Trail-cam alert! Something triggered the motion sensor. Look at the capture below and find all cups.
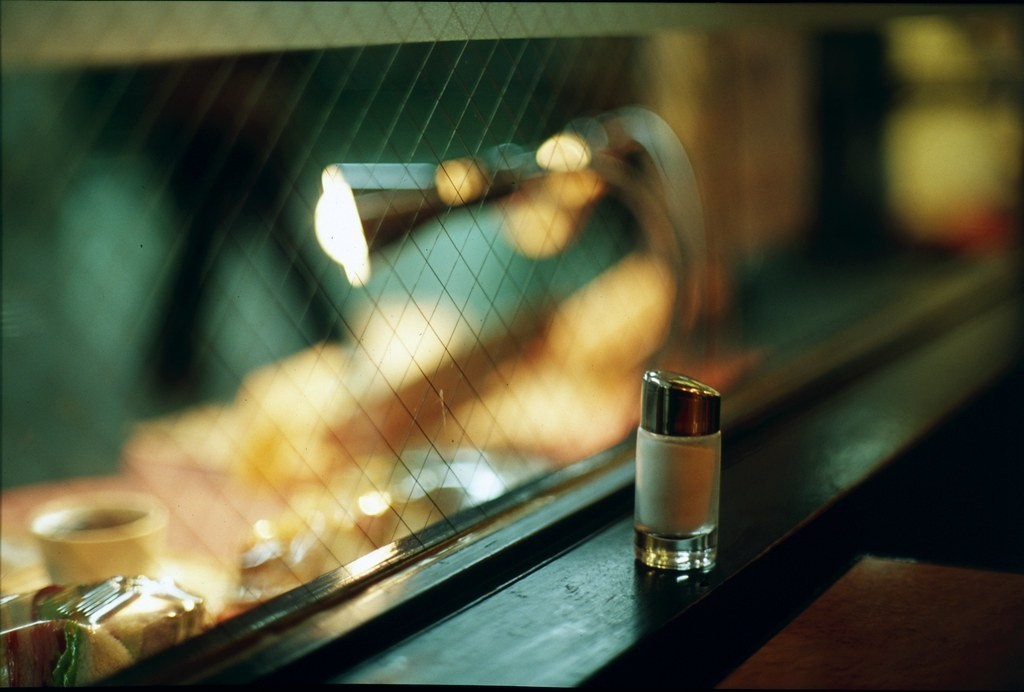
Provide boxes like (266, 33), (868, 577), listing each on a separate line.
(31, 506), (162, 586)
